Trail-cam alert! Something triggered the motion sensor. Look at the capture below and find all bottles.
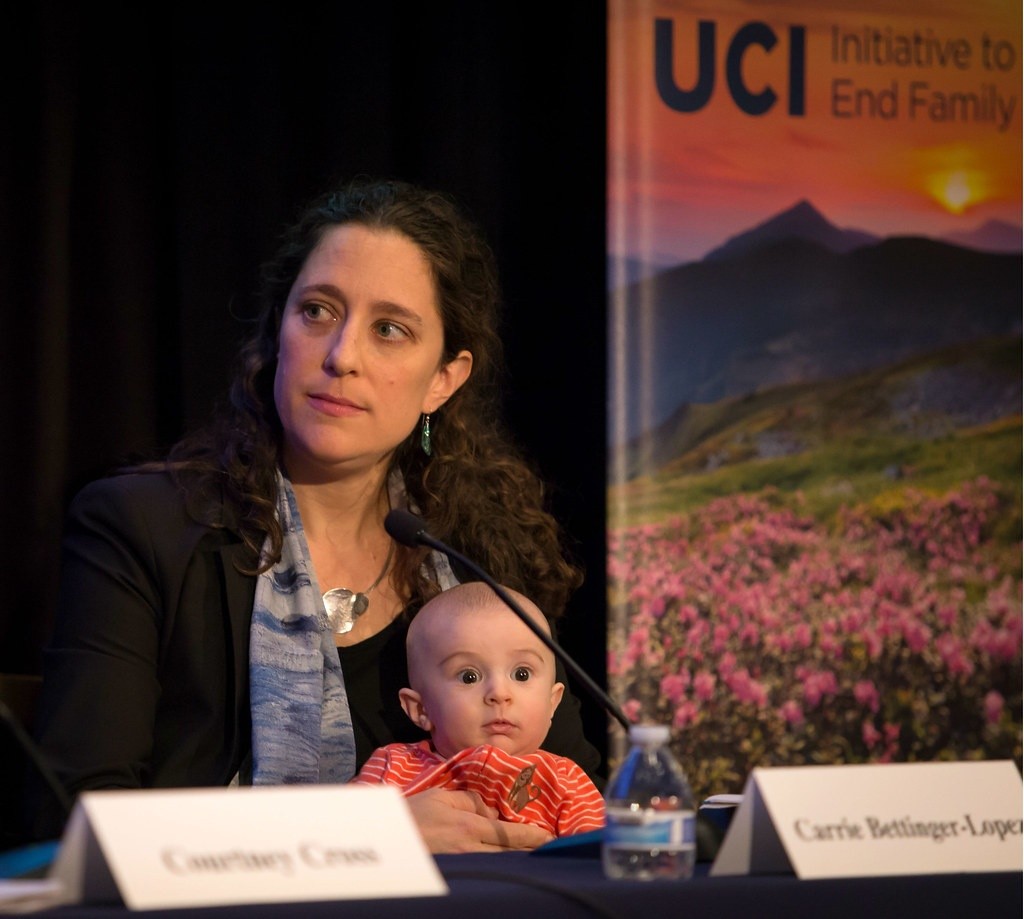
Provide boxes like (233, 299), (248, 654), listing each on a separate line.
(604, 724), (696, 883)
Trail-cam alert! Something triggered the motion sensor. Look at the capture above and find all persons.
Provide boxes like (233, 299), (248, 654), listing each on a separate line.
(345, 582), (607, 852)
(47, 180), (601, 806)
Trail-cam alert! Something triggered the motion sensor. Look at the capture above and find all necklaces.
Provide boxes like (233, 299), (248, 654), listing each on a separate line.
(322, 535), (407, 636)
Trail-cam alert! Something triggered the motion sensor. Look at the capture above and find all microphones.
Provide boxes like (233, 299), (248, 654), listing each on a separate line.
(382, 508), (719, 865)
